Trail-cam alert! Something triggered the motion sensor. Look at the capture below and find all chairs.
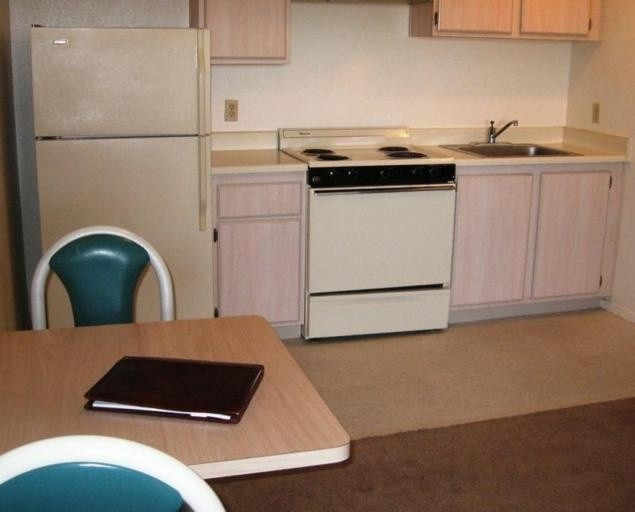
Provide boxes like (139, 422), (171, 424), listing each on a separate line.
(30, 225), (174, 331)
(0, 435), (232, 512)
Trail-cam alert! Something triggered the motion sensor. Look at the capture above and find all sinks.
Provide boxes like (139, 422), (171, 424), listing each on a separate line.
(438, 143), (584, 159)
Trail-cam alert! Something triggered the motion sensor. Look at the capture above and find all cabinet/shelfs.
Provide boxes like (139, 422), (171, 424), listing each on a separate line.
(408, 0), (603, 43)
(451, 160), (624, 310)
(211, 170), (305, 338)
(190, 0), (291, 65)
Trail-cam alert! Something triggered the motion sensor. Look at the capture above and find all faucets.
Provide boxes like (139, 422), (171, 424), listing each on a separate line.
(488, 119), (518, 144)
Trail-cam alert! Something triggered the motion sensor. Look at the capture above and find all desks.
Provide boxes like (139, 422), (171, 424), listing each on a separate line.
(0, 315), (351, 479)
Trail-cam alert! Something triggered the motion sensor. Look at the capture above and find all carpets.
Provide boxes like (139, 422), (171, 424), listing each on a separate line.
(181, 399), (635, 512)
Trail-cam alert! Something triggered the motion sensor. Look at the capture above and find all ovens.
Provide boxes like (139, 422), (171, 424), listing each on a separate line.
(304, 185), (456, 342)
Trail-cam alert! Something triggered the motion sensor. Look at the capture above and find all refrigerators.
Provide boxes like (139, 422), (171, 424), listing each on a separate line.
(29, 26), (215, 332)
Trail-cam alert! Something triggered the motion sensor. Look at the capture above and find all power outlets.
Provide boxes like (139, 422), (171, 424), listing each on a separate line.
(225, 99), (238, 122)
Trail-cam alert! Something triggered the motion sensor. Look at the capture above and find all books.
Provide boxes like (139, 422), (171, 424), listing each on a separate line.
(83, 355), (264, 424)
